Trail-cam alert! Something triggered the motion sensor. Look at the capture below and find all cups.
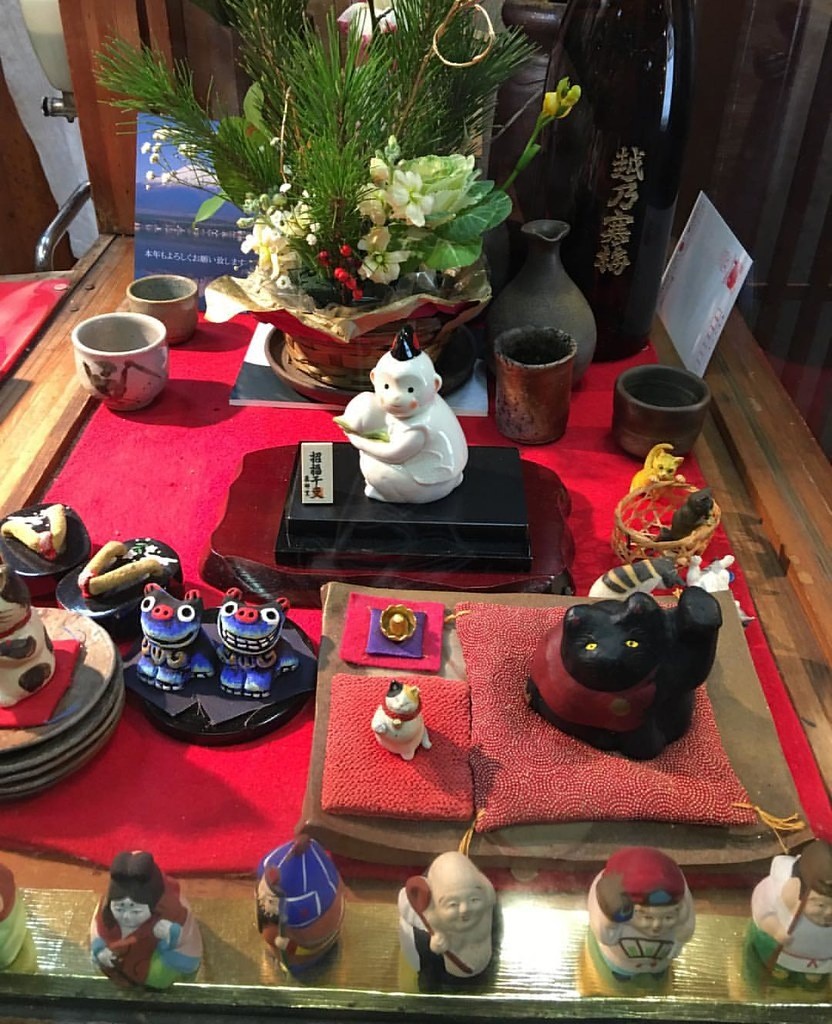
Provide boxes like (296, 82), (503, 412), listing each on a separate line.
(72, 313), (170, 412)
(613, 365), (709, 461)
(494, 329), (577, 445)
(126, 274), (199, 346)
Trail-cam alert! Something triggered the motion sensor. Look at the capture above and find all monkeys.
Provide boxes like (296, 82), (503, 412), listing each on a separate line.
(331, 324), (469, 505)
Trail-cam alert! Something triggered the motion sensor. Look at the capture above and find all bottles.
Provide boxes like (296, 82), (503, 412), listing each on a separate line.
(485, 220), (597, 385)
(539, 0), (692, 361)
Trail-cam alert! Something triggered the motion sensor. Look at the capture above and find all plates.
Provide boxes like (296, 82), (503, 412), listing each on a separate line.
(0, 609), (126, 802)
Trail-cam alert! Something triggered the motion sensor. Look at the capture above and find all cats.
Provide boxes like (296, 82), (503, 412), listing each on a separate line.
(588, 442), (756, 635)
(524, 585), (722, 761)
(0, 563), (57, 710)
(370, 678), (433, 761)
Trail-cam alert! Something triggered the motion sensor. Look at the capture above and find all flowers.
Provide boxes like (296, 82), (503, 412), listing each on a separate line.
(84, 0), (581, 306)
